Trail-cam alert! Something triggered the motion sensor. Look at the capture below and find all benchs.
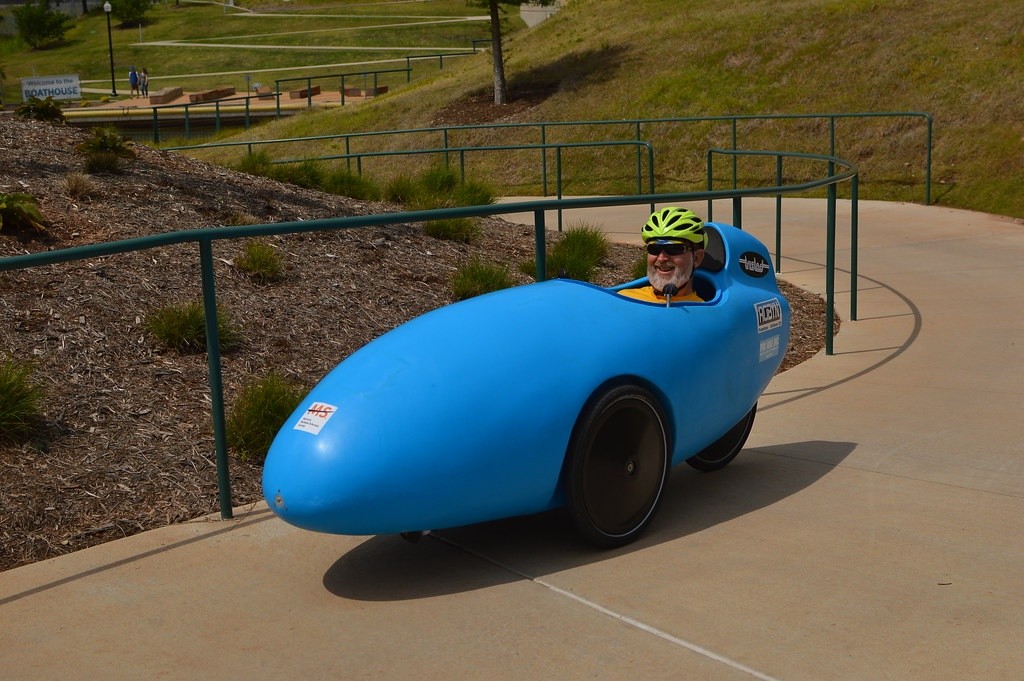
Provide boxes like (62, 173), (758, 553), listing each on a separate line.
(148, 86), (387, 106)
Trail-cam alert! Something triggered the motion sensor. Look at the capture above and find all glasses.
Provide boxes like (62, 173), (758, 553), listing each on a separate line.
(644, 243), (696, 256)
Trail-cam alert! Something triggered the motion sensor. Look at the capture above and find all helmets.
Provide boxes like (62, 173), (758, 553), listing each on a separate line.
(640, 206), (709, 251)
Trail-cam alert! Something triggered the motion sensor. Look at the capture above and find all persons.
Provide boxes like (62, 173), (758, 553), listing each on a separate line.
(128, 65), (148, 99)
(615, 206), (708, 304)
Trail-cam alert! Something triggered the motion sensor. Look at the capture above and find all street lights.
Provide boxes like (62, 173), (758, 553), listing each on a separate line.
(102, 1), (118, 96)
